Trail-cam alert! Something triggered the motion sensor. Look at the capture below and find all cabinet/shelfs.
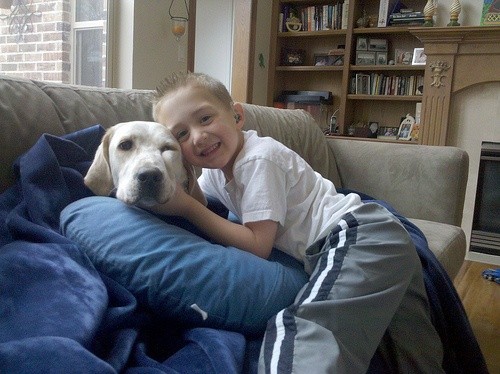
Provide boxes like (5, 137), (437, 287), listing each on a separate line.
(267, 0), (427, 144)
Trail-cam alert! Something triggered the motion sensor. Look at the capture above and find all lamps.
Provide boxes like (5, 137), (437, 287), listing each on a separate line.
(168, 0), (190, 42)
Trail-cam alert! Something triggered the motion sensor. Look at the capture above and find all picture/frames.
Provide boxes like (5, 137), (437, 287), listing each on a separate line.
(396, 113), (415, 141)
(412, 48), (427, 66)
(356, 74), (370, 95)
(313, 54), (330, 65)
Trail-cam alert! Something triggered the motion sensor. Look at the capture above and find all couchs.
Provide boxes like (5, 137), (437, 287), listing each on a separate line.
(0, 78), (466, 284)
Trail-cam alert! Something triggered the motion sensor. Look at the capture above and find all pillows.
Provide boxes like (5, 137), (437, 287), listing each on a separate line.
(59, 194), (308, 333)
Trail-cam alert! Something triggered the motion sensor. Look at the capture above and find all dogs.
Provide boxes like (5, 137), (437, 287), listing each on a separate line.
(83, 120), (209, 210)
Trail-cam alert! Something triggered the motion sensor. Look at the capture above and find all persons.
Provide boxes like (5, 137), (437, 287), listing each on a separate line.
(149, 69), (445, 374)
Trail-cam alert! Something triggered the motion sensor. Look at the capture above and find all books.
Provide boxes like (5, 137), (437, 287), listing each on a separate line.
(276, 2), (427, 130)
